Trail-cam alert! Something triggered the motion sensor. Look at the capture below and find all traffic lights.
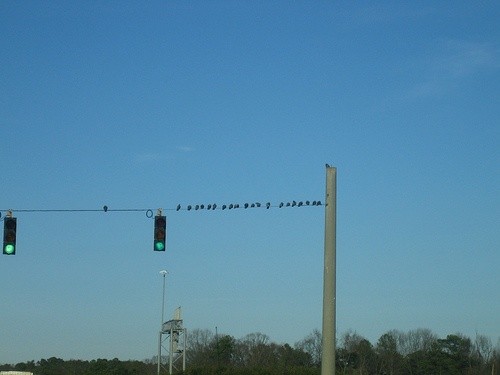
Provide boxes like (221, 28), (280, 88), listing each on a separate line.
(154, 216), (166, 250)
(3, 217), (16, 254)
